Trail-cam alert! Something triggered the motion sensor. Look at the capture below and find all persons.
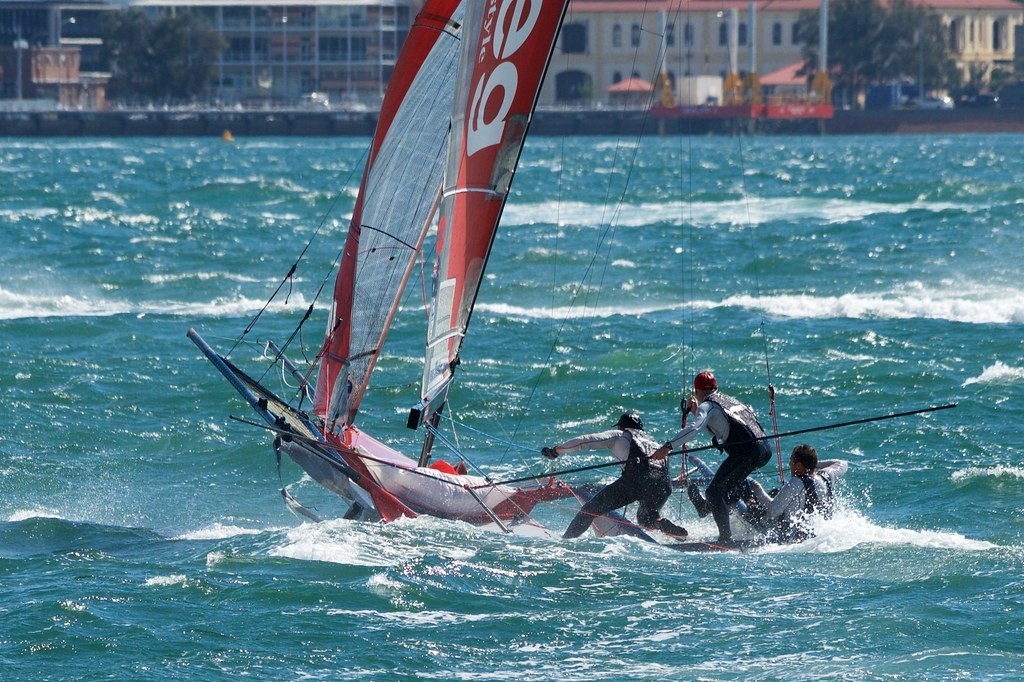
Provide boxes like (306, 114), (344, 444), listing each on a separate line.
(651, 372), (772, 548)
(541, 413), (688, 542)
(688, 444), (848, 545)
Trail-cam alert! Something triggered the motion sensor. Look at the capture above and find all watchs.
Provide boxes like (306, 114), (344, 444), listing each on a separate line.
(664, 442), (674, 450)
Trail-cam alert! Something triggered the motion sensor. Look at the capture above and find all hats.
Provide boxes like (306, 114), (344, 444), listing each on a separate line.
(611, 413), (645, 431)
(694, 371), (718, 390)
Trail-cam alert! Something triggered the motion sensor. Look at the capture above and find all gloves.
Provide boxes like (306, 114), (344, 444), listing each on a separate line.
(541, 446), (559, 460)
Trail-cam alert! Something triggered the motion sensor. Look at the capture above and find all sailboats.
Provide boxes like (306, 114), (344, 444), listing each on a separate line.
(185, 0), (847, 553)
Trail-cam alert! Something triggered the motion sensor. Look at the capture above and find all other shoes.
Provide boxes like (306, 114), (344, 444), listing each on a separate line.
(688, 484), (711, 518)
(659, 519), (688, 543)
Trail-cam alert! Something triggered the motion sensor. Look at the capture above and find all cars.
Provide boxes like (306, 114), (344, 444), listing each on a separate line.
(302, 94), (330, 109)
(906, 96), (958, 112)
